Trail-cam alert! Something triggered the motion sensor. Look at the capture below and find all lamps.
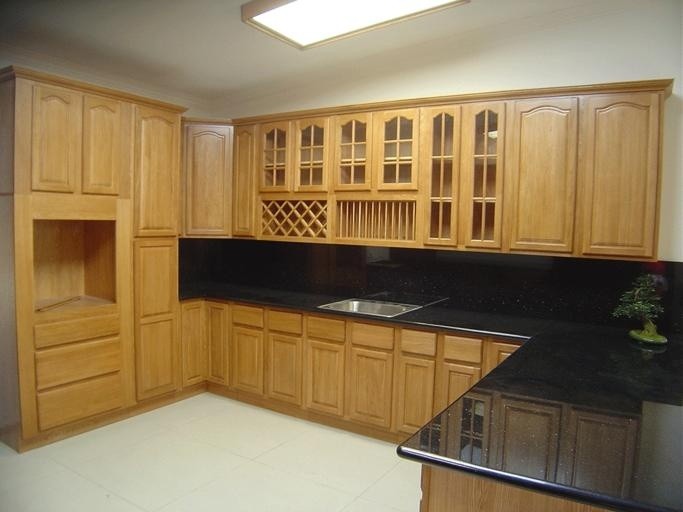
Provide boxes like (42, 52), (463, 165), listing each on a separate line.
(240, 1), (472, 52)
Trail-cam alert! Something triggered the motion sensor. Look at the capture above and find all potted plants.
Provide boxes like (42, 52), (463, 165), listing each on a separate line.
(613, 272), (670, 345)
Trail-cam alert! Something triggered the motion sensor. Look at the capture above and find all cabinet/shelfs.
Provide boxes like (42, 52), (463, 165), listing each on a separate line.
(395, 328), (441, 434)
(490, 340), (518, 372)
(262, 94), (500, 249)
(31, 84), (78, 192)
(177, 302), (204, 391)
(130, 103), (178, 238)
(417, 389), (492, 468)
(134, 238), (184, 401)
(504, 91), (661, 257)
(181, 118), (233, 242)
(438, 337), (485, 414)
(347, 325), (396, 432)
(211, 299), (229, 390)
(305, 317), (347, 422)
(229, 304), (266, 394)
(82, 90), (117, 193)
(229, 118), (257, 236)
(265, 307), (304, 412)
(495, 397), (638, 494)
(37, 219), (119, 440)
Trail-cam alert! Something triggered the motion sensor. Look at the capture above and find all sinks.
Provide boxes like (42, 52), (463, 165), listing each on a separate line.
(365, 291), (449, 307)
(318, 297), (424, 319)
